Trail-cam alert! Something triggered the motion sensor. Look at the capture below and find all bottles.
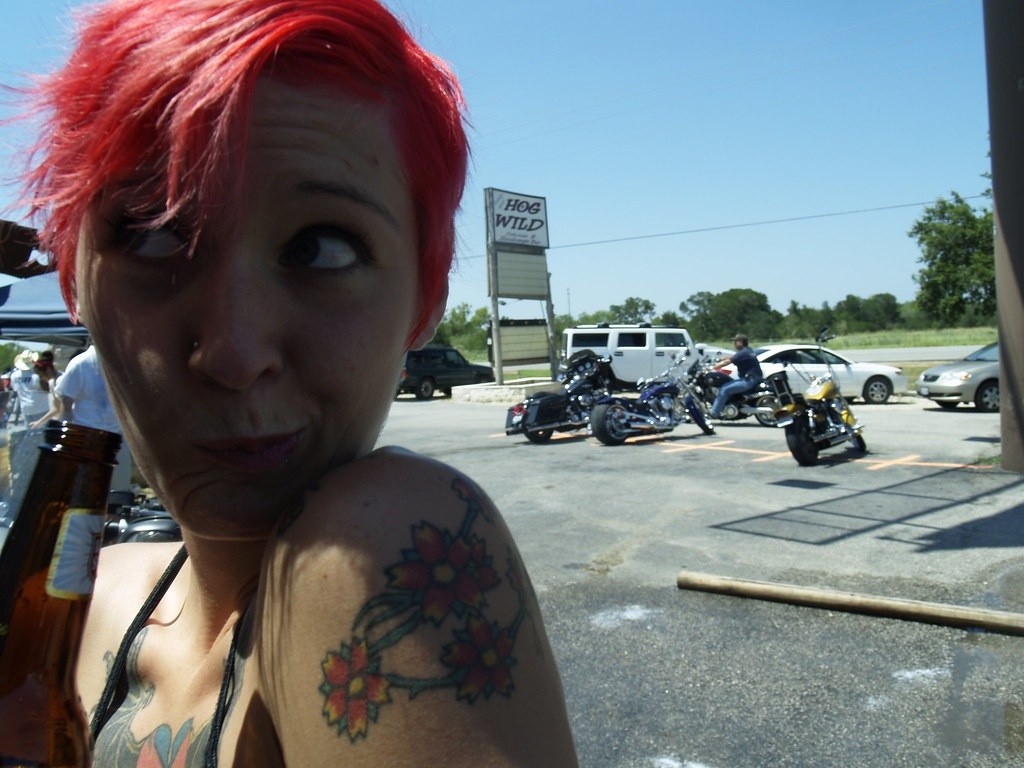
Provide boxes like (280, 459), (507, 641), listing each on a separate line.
(0, 420), (124, 768)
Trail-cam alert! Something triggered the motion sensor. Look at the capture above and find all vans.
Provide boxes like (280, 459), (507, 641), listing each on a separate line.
(556, 321), (704, 393)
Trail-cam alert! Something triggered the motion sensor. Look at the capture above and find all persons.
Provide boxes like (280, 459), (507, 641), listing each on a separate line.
(0, 330), (763, 492)
(0, 0), (576, 768)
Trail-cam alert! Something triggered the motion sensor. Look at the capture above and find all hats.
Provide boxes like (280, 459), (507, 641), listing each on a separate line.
(13, 350), (39, 371)
(731, 335), (747, 342)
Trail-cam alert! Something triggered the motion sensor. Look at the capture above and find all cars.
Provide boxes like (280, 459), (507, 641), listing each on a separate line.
(914, 340), (1002, 413)
(714, 342), (909, 406)
(696, 342), (738, 368)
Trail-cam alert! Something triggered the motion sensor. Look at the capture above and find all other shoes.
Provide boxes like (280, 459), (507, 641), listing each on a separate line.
(703, 408), (714, 419)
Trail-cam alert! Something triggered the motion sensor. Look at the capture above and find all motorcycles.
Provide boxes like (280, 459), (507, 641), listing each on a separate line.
(591, 348), (716, 444)
(776, 328), (868, 466)
(681, 349), (781, 428)
(504, 347), (615, 445)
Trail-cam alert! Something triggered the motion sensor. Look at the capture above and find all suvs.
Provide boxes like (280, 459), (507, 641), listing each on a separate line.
(394, 345), (495, 400)
